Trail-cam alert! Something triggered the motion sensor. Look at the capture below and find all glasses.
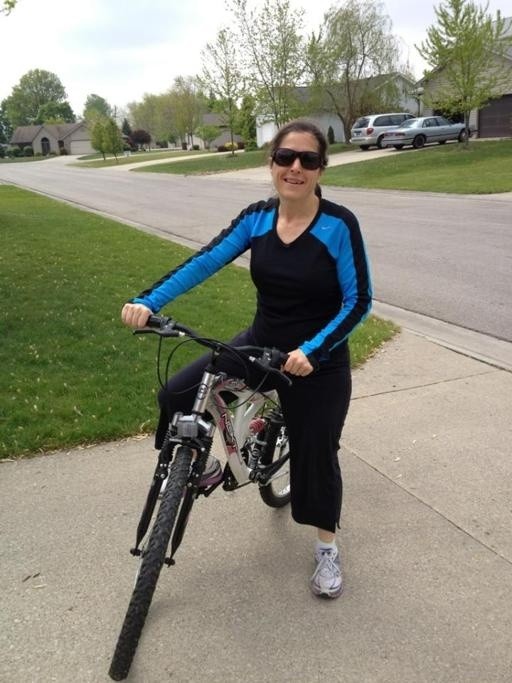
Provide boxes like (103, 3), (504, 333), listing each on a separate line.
(272, 148), (322, 170)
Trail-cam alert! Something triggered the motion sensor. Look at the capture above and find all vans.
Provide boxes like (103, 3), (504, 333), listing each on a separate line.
(348, 112), (416, 150)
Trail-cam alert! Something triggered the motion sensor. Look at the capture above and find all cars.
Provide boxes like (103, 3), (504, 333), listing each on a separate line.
(381, 115), (472, 149)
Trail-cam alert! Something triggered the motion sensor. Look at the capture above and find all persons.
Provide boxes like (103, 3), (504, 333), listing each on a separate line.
(118, 114), (375, 600)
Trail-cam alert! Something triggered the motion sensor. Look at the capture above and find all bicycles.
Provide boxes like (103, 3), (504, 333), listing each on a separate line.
(108, 313), (292, 682)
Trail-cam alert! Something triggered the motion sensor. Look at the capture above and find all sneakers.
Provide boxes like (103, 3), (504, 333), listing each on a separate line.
(310, 546), (343, 600)
(158, 456), (222, 500)
